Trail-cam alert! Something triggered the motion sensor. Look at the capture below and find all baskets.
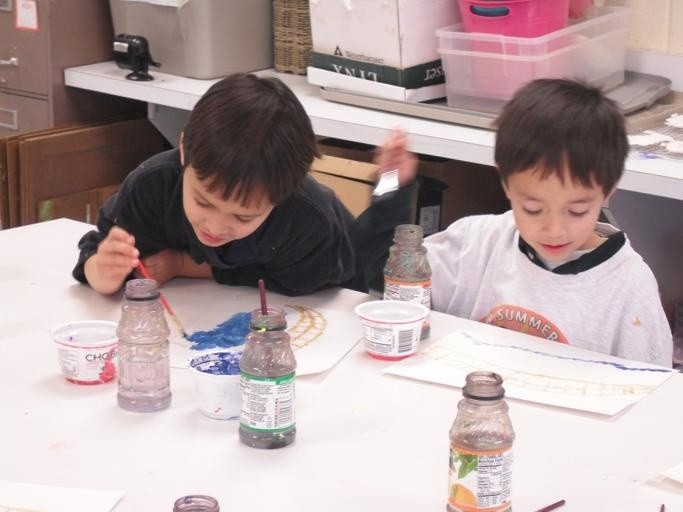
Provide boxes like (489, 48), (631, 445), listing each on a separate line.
(273, 1), (313, 74)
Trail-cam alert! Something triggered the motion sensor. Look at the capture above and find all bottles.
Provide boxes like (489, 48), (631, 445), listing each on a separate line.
(383, 222), (434, 342)
(446, 369), (518, 512)
(117, 277), (173, 413)
(237, 306), (299, 450)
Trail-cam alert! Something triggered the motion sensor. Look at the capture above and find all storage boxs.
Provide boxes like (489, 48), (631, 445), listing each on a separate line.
(109, 0), (274, 80)
(307, 137), (450, 235)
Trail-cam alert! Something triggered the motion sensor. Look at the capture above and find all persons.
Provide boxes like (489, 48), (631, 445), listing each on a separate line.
(351, 79), (672, 367)
(73, 72), (368, 296)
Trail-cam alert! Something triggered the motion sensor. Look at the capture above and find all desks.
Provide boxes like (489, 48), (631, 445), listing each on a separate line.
(0, 216), (683, 511)
(62, 59), (683, 201)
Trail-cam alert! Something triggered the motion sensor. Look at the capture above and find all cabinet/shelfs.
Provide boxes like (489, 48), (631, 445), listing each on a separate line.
(0, 0), (130, 135)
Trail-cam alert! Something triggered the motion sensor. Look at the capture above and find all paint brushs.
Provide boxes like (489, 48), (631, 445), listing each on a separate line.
(137, 259), (190, 343)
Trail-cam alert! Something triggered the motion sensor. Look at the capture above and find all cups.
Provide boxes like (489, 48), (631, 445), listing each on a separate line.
(190, 350), (250, 420)
(52, 318), (120, 385)
(354, 297), (429, 359)
